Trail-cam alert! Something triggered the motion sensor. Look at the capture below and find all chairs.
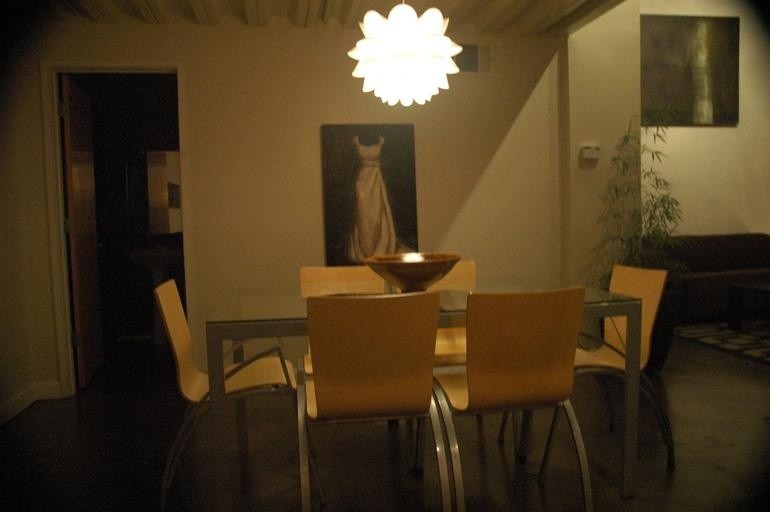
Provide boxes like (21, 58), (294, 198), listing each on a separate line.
(299, 261), (477, 316)
(498, 263), (678, 488)
(295, 289), (452, 510)
(432, 286), (596, 509)
(154, 277), (328, 510)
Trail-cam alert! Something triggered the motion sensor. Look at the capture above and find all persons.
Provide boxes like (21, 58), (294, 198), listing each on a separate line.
(335, 127), (416, 264)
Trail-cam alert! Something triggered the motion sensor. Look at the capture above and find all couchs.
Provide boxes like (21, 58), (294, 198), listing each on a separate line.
(641, 233), (770, 321)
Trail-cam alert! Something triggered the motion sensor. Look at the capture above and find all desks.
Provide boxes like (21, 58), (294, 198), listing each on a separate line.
(206, 285), (641, 508)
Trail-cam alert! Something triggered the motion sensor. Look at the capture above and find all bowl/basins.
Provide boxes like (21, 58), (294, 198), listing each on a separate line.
(367, 252), (462, 295)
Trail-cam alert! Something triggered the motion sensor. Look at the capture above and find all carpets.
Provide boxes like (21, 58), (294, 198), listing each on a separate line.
(674, 320), (770, 366)
